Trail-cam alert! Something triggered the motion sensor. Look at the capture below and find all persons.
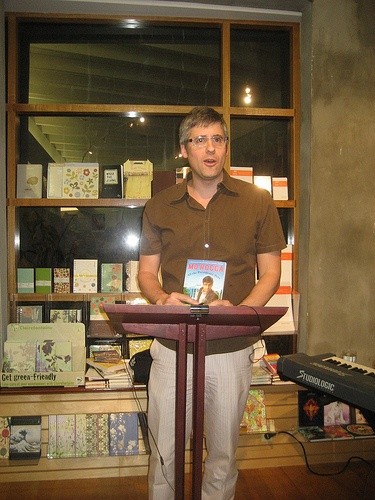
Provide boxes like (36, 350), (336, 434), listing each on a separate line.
(136, 105), (283, 500)
(193, 275), (219, 305)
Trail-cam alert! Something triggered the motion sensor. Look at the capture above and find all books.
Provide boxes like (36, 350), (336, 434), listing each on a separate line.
(0, 243), (294, 461)
(16, 159), (289, 201)
(182, 258), (229, 306)
(296, 389), (375, 442)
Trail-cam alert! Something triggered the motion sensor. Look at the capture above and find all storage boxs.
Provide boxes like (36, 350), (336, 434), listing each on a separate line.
(17, 161), (293, 294)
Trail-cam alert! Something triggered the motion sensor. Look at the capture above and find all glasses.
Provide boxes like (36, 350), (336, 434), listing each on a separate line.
(184, 135), (228, 148)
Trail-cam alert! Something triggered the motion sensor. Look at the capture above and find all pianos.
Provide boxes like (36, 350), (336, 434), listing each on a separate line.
(277, 353), (375, 433)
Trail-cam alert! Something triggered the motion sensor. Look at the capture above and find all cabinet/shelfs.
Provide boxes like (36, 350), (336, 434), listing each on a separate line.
(4, 9), (304, 393)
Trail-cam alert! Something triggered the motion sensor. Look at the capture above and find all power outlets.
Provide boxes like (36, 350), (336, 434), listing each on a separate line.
(263, 432), (274, 443)
